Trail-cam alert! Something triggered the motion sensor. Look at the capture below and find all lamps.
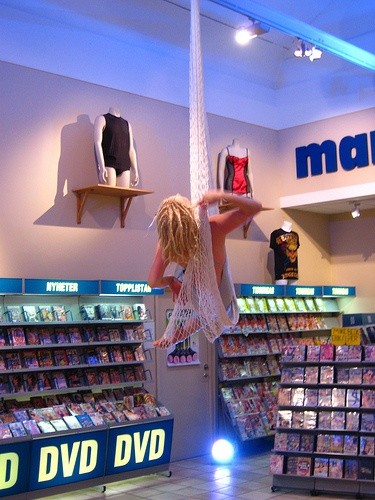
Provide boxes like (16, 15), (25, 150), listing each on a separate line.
(294, 47), (323, 62)
(235, 22), (270, 45)
(351, 203), (361, 219)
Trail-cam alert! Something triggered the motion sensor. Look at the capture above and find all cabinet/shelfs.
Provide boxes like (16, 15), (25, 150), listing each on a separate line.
(0, 296), (375, 500)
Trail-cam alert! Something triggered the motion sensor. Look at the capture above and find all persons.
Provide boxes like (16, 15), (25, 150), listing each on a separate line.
(148, 191), (263, 348)
(219, 140), (254, 205)
(94, 106), (139, 188)
(270, 221), (301, 285)
